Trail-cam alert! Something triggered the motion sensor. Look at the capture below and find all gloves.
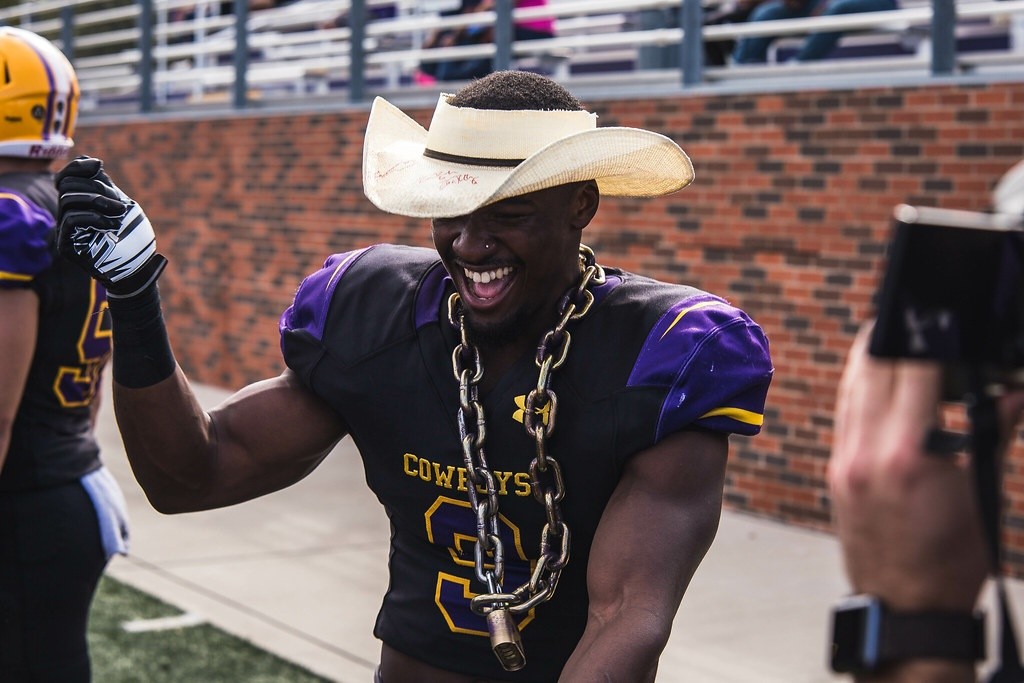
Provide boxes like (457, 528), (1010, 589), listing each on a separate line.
(54, 154), (166, 298)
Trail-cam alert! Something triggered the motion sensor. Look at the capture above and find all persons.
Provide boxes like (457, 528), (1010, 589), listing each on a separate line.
(830, 158), (1024, 683)
(53, 70), (771, 683)
(169, 0), (900, 84)
(0, 25), (131, 683)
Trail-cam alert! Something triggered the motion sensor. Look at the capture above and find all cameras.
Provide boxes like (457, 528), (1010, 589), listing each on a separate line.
(867, 204), (1024, 405)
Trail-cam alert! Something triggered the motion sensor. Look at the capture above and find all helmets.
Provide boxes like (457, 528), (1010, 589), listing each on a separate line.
(0, 22), (80, 160)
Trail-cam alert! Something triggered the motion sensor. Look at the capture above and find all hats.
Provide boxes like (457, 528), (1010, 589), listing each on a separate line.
(363, 92), (694, 218)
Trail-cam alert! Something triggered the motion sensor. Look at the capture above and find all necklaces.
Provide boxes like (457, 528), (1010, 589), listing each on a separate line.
(440, 243), (606, 671)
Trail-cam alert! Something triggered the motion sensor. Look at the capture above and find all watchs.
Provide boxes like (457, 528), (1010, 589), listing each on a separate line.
(831, 596), (986, 675)
